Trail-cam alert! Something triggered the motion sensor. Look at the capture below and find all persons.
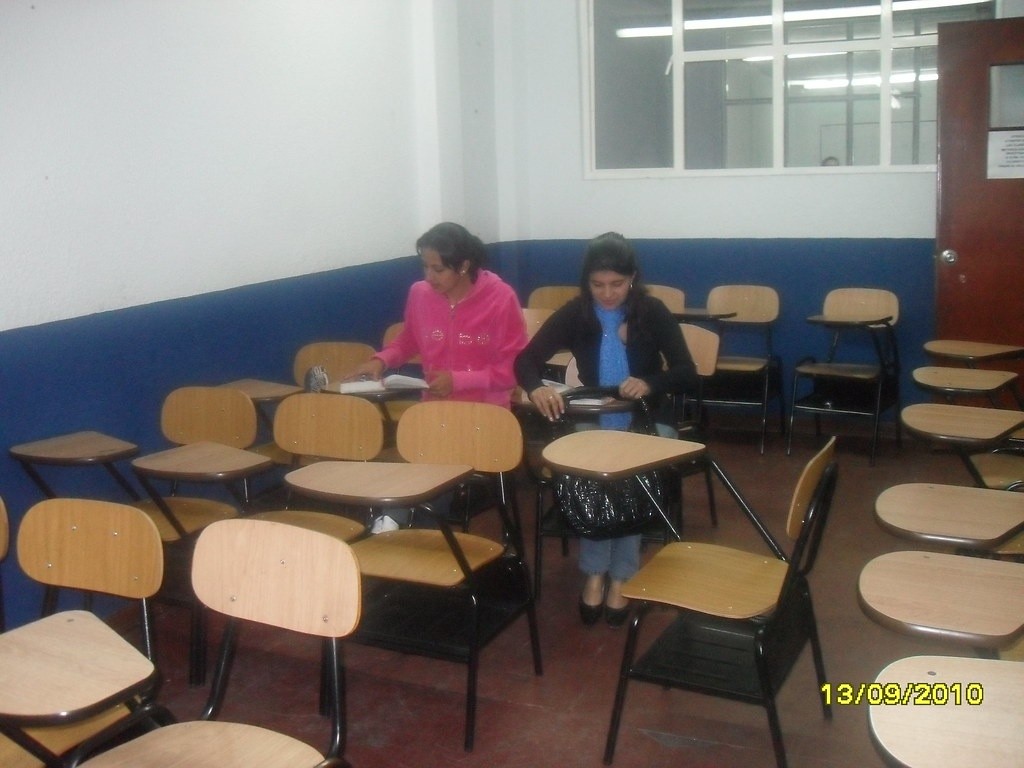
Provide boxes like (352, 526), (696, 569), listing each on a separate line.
(344, 223), (529, 535)
(514, 231), (697, 629)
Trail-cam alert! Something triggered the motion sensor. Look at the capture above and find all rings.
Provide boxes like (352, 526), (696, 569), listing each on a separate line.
(549, 396), (553, 399)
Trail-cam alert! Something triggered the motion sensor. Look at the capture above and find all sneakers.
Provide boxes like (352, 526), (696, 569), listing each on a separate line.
(371, 515), (400, 536)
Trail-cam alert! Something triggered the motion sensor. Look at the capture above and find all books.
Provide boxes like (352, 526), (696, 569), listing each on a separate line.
(341, 375), (431, 394)
(540, 378), (615, 406)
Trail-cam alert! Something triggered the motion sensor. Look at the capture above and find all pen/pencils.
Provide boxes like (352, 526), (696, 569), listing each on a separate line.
(360, 373), (366, 382)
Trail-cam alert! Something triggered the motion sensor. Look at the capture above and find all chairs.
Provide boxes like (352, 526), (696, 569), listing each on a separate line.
(0, 277), (1023, 768)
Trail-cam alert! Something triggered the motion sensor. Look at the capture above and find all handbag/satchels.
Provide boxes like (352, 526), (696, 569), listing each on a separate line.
(551, 385), (681, 541)
(669, 419), (716, 469)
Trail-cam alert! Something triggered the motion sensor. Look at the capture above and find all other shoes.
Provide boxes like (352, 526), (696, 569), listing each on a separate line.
(578, 584), (606, 626)
(606, 586), (632, 629)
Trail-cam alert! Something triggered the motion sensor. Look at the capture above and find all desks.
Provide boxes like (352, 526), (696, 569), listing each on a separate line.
(541, 428), (707, 479)
(664, 304), (739, 322)
(129, 439), (277, 483)
(283, 459), (475, 508)
(512, 392), (635, 414)
(901, 403), (1023, 450)
(213, 375), (304, 403)
(71, 719), (351, 768)
(921, 339), (1024, 363)
(855, 548), (1024, 649)
(0, 606), (164, 728)
(864, 654), (1024, 767)
(806, 316), (892, 325)
(869, 482), (1024, 549)
(318, 375), (422, 401)
(10, 428), (138, 464)
(910, 365), (1020, 396)
